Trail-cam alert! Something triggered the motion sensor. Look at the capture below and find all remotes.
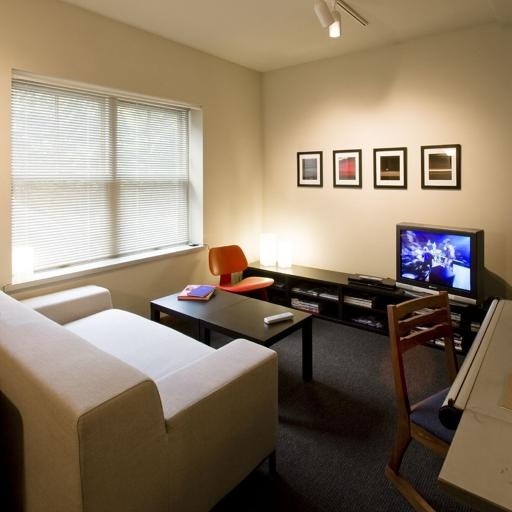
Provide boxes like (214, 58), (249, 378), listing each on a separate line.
(264, 312), (294, 325)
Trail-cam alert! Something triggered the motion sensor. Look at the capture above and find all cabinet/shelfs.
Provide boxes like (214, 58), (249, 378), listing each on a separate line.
(241, 257), (490, 356)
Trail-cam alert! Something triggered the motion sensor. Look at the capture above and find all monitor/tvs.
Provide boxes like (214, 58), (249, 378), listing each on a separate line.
(396, 222), (484, 305)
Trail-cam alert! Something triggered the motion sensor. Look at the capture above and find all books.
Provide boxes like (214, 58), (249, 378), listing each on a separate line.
(177, 284), (216, 301)
(187, 285), (216, 298)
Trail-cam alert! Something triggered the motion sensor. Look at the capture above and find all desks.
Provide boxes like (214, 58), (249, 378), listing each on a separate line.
(437, 298), (512, 511)
(149, 287), (316, 383)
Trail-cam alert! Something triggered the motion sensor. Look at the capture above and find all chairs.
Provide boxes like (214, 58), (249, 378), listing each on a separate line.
(383, 288), (461, 512)
(2, 280), (285, 512)
(207, 243), (276, 303)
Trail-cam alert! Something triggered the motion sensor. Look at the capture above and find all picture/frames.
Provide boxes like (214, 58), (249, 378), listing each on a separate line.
(372, 147), (408, 191)
(296, 150), (324, 188)
(419, 144), (462, 192)
(332, 149), (363, 189)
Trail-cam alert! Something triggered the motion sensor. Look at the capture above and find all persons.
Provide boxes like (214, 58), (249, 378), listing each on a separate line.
(420, 246), (434, 270)
(442, 240), (455, 266)
